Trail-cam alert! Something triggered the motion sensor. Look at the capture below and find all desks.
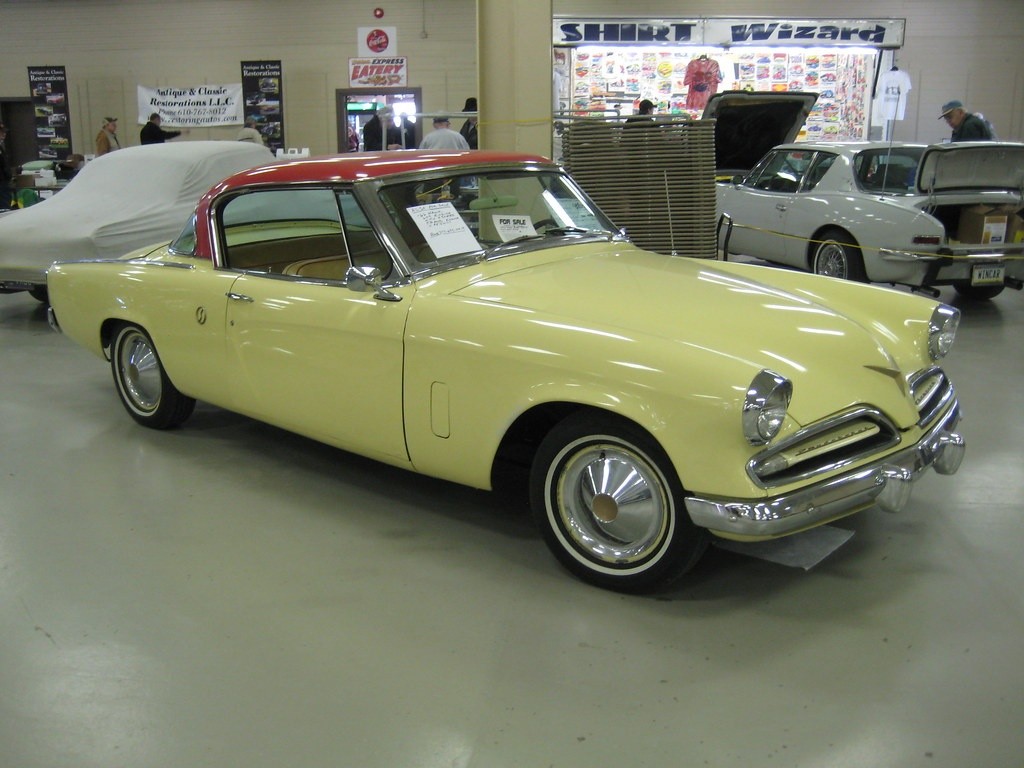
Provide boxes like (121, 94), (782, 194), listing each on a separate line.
(6, 175), (73, 203)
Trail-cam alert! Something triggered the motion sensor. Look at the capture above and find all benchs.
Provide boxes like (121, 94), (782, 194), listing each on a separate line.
(228, 233), (488, 281)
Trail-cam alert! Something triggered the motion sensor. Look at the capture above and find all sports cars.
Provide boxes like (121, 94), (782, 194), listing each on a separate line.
(45, 147), (966, 599)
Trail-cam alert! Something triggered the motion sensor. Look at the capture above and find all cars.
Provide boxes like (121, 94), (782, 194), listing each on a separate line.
(247, 77), (282, 151)
(34, 83), (69, 158)
(700, 88), (1024, 301)
(0, 138), (333, 306)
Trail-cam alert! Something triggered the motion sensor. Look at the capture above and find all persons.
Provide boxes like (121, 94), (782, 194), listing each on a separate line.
(238, 116), (265, 145)
(140, 113), (181, 145)
(96, 117), (121, 157)
(620, 100), (664, 143)
(0, 123), (11, 182)
(938, 101), (999, 144)
(364, 98), (479, 194)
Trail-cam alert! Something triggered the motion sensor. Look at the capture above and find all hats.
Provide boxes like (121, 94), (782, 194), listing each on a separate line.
(462, 97), (477, 112)
(245, 117), (255, 124)
(433, 111), (449, 121)
(639, 99), (657, 108)
(938, 100), (963, 119)
(102, 117), (118, 128)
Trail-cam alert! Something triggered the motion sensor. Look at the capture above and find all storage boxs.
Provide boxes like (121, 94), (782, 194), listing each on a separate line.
(956, 205), (1008, 244)
(986, 204), (1024, 244)
